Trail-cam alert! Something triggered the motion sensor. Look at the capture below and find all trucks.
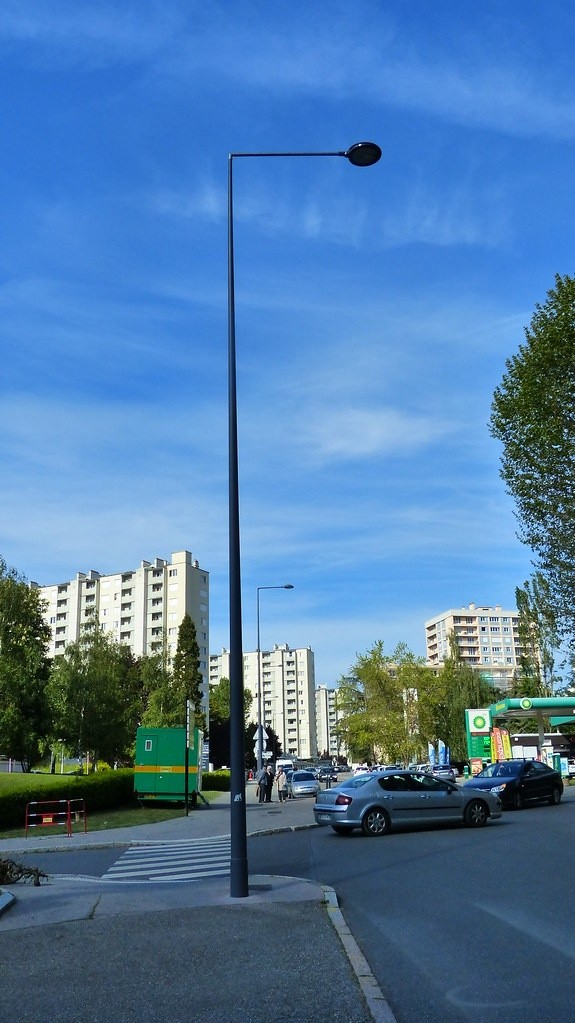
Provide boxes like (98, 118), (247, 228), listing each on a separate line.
(275, 759), (296, 775)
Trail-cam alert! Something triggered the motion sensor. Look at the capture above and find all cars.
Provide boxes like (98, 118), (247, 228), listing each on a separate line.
(287, 767), (318, 780)
(463, 757), (563, 810)
(319, 767), (337, 782)
(290, 772), (321, 798)
(334, 766), (350, 772)
(313, 770), (502, 836)
(351, 767), (370, 776)
(426, 764), (455, 782)
(371, 763), (416, 772)
(416, 764), (431, 779)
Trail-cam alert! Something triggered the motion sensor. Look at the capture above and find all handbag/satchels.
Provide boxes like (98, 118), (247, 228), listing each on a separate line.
(255, 784), (260, 797)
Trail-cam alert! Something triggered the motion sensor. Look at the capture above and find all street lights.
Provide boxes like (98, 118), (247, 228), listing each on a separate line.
(255, 584), (294, 772)
(222, 143), (384, 898)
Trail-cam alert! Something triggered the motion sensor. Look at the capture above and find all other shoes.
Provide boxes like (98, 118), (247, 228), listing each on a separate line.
(266, 800), (273, 802)
(278, 800), (282, 802)
(283, 800), (285, 802)
(259, 800), (265, 803)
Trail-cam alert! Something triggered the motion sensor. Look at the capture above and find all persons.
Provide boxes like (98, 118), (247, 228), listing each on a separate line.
(457, 760), (470, 777)
(275, 767), (287, 803)
(500, 766), (509, 776)
(257, 766), (273, 803)
(424, 764), (433, 785)
